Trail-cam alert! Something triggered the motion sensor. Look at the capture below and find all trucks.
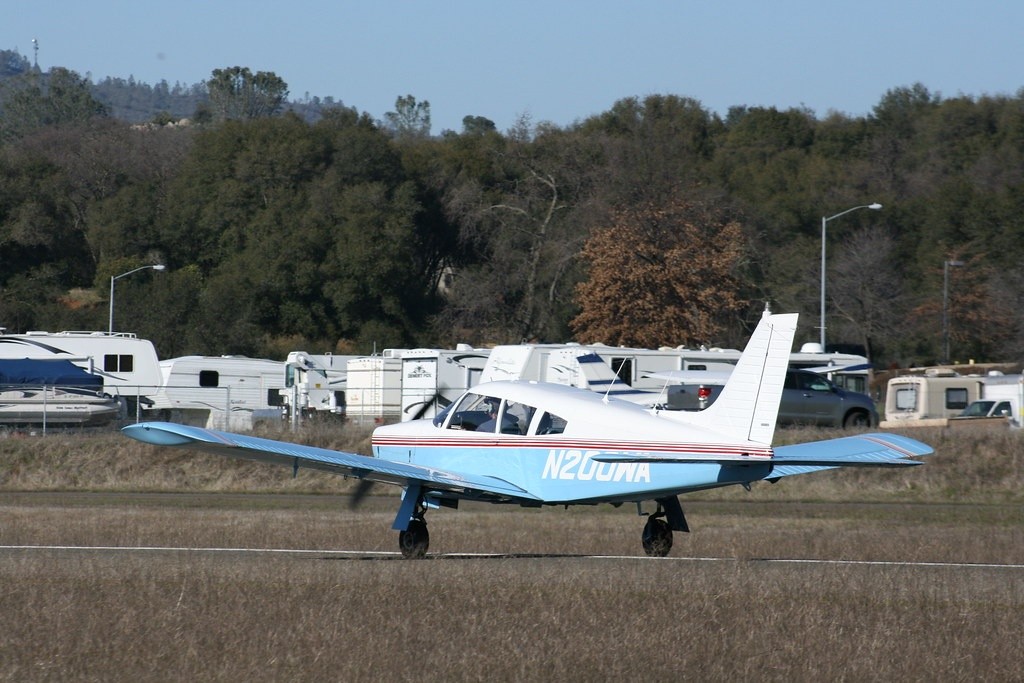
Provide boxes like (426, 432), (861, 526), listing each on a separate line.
(956, 374), (1024, 431)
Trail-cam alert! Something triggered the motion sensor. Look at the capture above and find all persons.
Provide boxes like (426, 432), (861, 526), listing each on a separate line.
(475, 399), (518, 434)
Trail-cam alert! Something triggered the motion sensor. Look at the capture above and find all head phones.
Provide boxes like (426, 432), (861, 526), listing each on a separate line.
(487, 403), (493, 413)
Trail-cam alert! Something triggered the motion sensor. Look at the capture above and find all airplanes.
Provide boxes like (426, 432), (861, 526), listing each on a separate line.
(119, 301), (936, 560)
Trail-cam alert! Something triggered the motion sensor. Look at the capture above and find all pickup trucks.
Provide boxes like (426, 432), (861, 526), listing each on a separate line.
(663, 367), (880, 432)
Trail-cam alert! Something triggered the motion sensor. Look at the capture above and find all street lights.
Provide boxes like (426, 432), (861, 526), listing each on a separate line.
(820, 202), (885, 352)
(108, 263), (165, 336)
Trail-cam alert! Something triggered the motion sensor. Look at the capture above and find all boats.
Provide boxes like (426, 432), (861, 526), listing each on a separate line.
(0, 357), (123, 429)
